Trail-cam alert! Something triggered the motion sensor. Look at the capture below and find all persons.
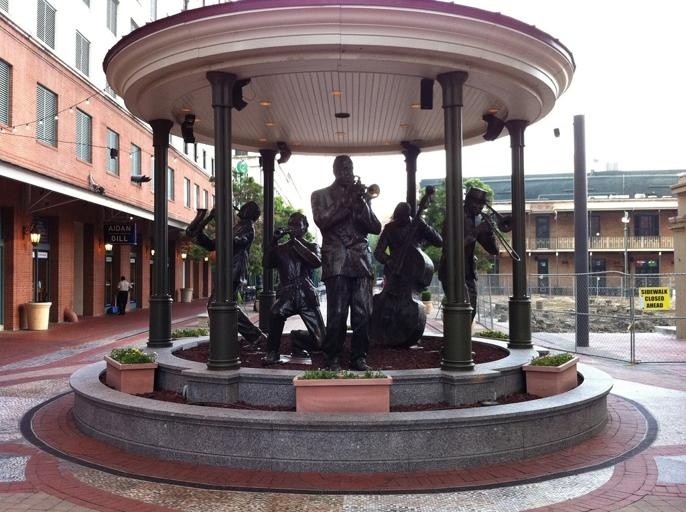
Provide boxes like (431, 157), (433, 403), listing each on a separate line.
(193, 201), (267, 351)
(260, 213), (326, 361)
(438, 186), (499, 357)
(117, 276), (133, 316)
(311, 155), (381, 370)
(374, 202), (443, 275)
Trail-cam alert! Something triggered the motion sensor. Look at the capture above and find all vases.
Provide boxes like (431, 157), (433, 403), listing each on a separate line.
(104, 354), (158, 395)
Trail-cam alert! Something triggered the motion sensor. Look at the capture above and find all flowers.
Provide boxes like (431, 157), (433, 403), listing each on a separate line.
(111, 346), (158, 364)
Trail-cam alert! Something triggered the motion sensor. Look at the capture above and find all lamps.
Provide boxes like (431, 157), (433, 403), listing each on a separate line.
(177, 248), (187, 260)
(99, 237), (114, 252)
(204, 256), (208, 264)
(23, 223), (41, 246)
(146, 246), (155, 255)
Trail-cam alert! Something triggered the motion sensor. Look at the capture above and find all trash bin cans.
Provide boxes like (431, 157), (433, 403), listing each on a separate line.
(25, 302), (52, 331)
(180, 288), (193, 303)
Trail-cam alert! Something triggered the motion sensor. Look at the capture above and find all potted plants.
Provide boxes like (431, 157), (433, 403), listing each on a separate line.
(522, 351), (580, 396)
(292, 368), (393, 413)
